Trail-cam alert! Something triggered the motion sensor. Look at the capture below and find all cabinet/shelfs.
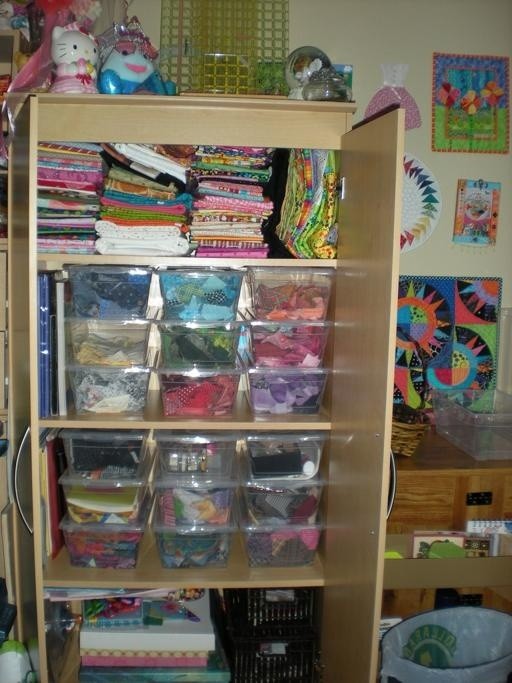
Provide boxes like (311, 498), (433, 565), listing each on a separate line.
(1, 93), (409, 682)
(383, 424), (511, 620)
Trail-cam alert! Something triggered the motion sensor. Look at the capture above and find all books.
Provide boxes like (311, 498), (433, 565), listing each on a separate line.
(384, 519), (512, 558)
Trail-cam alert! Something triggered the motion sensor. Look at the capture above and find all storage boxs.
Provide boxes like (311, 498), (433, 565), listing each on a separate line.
(429, 382), (512, 462)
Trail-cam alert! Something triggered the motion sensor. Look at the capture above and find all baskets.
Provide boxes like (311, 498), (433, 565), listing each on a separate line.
(226, 625), (316, 682)
(224, 587), (317, 631)
(391, 402), (431, 457)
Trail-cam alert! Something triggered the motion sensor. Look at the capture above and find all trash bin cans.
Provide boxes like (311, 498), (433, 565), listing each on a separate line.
(380, 607), (512, 683)
